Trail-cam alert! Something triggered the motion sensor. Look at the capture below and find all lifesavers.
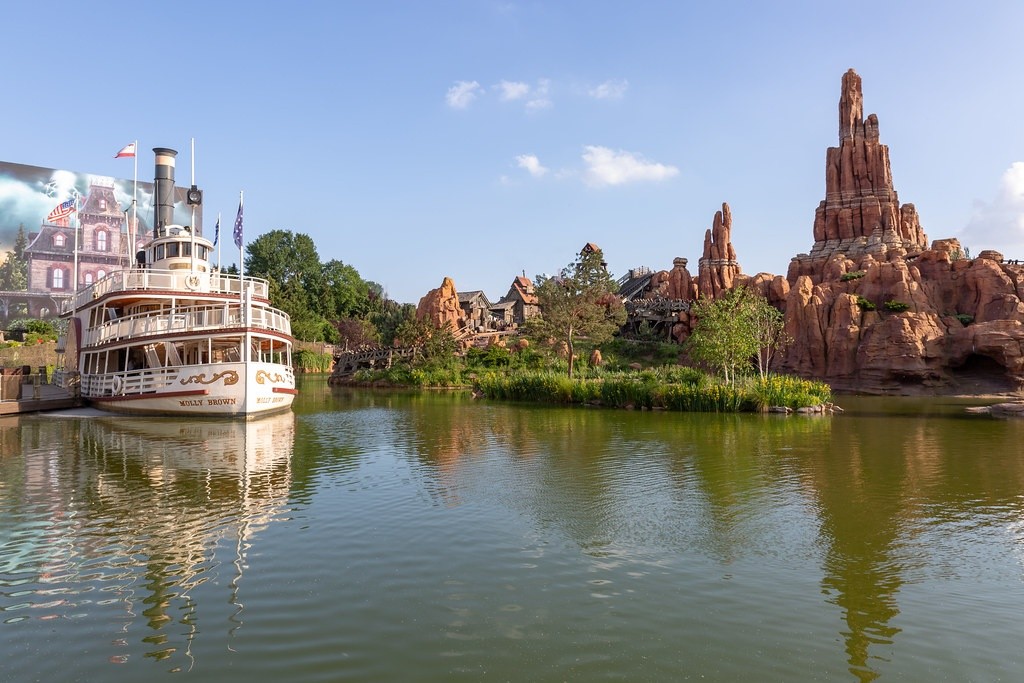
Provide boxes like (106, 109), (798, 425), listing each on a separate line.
(112, 376), (121, 394)
(185, 274), (202, 290)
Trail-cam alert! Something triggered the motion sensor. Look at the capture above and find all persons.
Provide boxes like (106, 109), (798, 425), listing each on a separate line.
(128, 359), (136, 377)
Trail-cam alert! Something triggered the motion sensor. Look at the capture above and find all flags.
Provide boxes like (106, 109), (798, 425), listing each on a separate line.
(114, 140), (138, 158)
(214, 212), (221, 246)
(48, 195), (79, 222)
(233, 190), (244, 248)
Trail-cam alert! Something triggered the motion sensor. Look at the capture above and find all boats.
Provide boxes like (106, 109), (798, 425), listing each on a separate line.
(51, 138), (300, 420)
